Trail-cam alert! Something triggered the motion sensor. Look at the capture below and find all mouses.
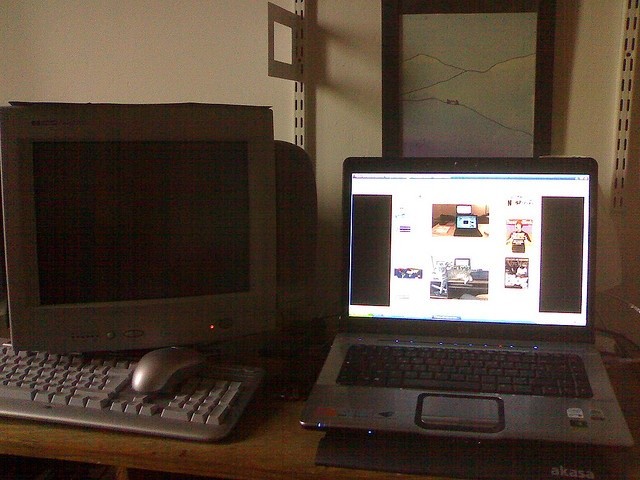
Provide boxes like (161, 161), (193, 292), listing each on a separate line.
(132, 348), (207, 395)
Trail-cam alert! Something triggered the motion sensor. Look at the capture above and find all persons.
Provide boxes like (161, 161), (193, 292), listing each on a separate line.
(506, 221), (531, 253)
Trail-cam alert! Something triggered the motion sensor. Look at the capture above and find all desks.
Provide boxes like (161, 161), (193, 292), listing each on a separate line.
(0, 312), (636, 478)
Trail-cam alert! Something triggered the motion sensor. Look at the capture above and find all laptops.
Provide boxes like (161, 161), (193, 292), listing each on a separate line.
(299, 157), (634, 450)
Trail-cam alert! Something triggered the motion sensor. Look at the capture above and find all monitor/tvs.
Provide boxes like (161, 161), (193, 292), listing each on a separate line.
(0, 106), (277, 353)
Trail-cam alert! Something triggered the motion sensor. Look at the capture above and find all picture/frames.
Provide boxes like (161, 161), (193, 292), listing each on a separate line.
(380, 0), (556, 158)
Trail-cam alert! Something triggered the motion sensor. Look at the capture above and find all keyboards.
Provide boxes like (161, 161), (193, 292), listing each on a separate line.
(0, 345), (266, 440)
